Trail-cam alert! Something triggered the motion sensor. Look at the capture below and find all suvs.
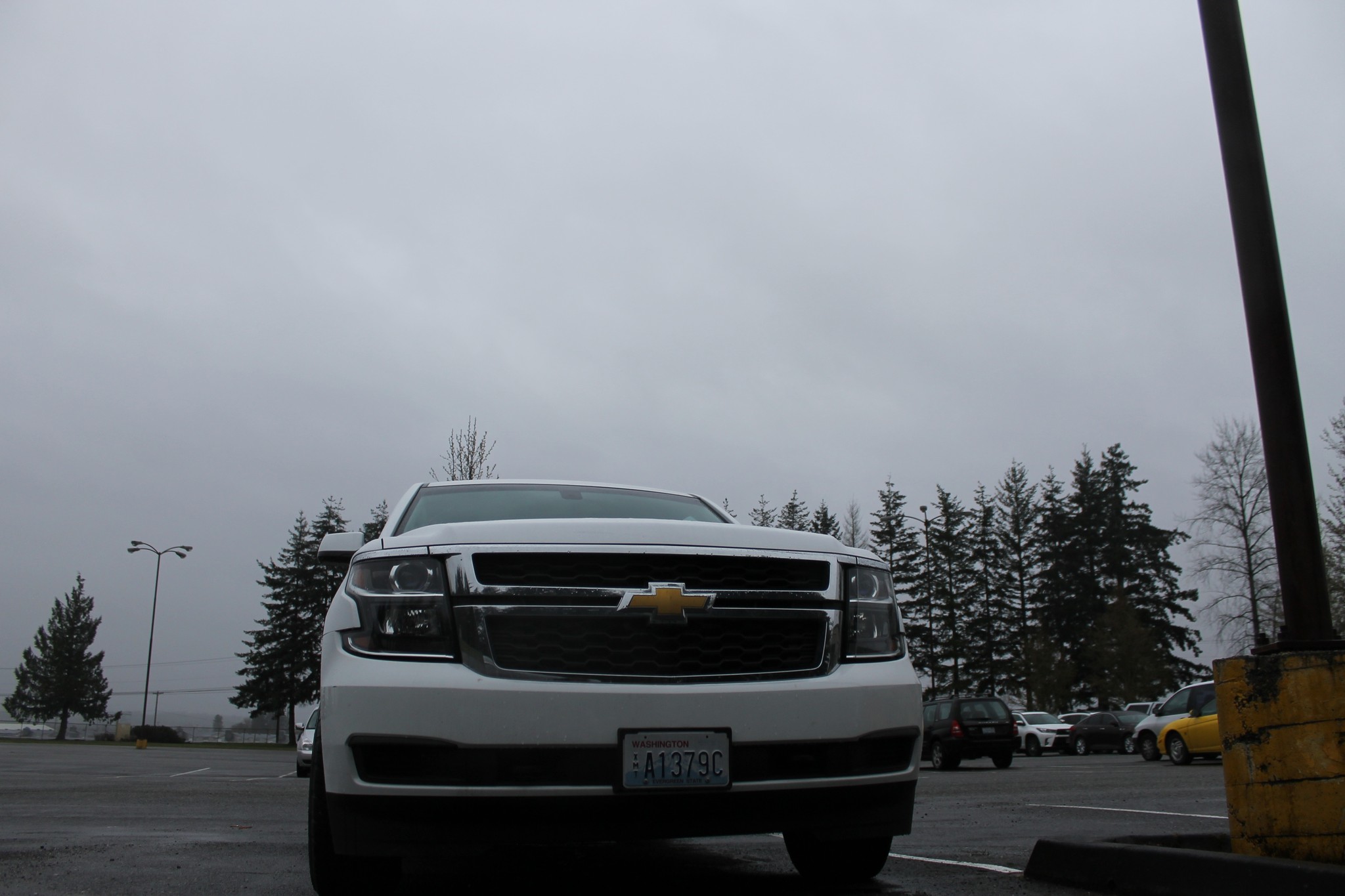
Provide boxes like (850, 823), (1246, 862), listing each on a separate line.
(920, 693), (1018, 771)
(307, 478), (923, 896)
(1124, 680), (1215, 761)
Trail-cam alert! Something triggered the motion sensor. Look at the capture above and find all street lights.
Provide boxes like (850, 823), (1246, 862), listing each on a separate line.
(881, 505), (973, 702)
(128, 541), (193, 726)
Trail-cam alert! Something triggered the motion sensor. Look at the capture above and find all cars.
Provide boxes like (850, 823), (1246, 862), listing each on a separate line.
(1058, 710), (1150, 755)
(1010, 712), (1072, 755)
(1156, 697), (1223, 765)
(296, 708), (319, 776)
